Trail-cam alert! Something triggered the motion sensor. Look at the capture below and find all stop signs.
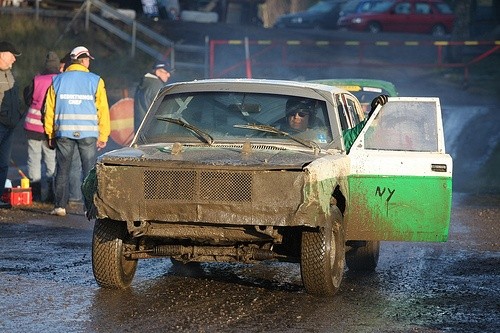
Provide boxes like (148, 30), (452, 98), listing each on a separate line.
(109, 98), (135, 148)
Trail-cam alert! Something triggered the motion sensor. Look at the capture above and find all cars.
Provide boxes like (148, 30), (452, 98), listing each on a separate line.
(335, 0), (456, 35)
(271, 0), (377, 35)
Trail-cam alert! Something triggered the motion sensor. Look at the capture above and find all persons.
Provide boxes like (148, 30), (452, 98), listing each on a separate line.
(263, 94), (389, 155)
(43, 46), (111, 217)
(134, 62), (171, 136)
(0, 41), (23, 199)
(24, 51), (64, 203)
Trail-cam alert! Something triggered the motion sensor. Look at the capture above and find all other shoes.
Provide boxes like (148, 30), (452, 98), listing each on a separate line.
(0, 199), (10, 208)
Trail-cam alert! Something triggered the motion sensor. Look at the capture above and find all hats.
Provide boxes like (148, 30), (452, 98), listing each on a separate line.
(0, 41), (21, 56)
(44, 51), (60, 68)
(152, 59), (176, 73)
(69, 46), (95, 60)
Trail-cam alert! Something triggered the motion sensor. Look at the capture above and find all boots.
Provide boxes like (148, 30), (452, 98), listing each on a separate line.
(31, 182), (41, 202)
(45, 176), (56, 203)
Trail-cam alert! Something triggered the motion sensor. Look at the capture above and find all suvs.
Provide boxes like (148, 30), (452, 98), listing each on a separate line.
(81, 79), (453, 295)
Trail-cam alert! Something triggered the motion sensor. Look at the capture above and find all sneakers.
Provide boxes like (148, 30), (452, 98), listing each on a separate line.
(50, 207), (67, 216)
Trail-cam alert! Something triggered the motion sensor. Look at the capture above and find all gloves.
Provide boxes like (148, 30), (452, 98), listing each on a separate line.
(368, 94), (389, 117)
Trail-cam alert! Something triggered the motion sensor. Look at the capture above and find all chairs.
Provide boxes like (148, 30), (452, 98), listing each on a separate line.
(269, 115), (325, 140)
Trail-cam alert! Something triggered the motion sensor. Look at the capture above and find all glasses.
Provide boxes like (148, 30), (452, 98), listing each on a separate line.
(286, 111), (310, 117)
